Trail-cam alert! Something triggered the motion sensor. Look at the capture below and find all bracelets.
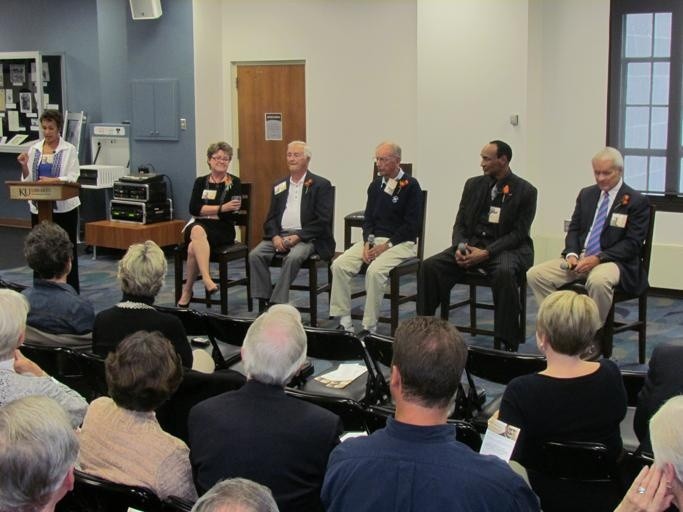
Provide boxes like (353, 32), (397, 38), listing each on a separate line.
(218, 204), (222, 213)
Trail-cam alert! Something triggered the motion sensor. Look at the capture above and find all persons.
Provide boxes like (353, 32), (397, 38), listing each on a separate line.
(320, 315), (540, 510)
(248, 139), (335, 307)
(330, 142), (421, 341)
(73, 331), (198, 503)
(92, 240), (215, 374)
(0, 397), (81, 511)
(20, 223), (95, 335)
(177, 142), (245, 308)
(525, 145), (649, 363)
(187, 304), (341, 509)
(615, 395), (682, 510)
(192, 477), (278, 510)
(494, 291), (642, 510)
(633, 346), (682, 455)
(499, 425), (516, 439)
(419, 142), (533, 350)
(2, 289), (89, 429)
(18, 109), (81, 296)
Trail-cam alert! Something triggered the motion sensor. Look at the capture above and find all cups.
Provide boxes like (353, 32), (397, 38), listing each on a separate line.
(232, 195), (242, 214)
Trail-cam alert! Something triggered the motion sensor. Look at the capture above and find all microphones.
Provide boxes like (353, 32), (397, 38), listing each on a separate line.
(276, 239), (290, 253)
(458, 243), (488, 277)
(368, 234), (375, 263)
(560, 260), (578, 272)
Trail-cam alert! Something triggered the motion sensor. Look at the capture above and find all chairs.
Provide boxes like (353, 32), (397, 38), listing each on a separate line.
(351, 190), (428, 338)
(440, 230), (530, 351)
(174, 182), (254, 315)
(344, 164), (412, 275)
(557, 205), (657, 365)
(258, 186), (336, 327)
(2, 282), (682, 512)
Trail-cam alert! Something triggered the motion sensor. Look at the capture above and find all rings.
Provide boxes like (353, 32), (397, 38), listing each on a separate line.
(640, 487), (644, 493)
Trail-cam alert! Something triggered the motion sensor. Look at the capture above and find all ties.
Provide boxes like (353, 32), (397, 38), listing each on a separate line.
(490, 184), (498, 201)
(584, 191), (610, 257)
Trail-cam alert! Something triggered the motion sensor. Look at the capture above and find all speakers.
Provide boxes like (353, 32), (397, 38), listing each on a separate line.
(129, 0), (163, 20)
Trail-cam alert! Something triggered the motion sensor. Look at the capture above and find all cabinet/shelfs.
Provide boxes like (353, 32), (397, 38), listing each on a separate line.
(132, 77), (180, 142)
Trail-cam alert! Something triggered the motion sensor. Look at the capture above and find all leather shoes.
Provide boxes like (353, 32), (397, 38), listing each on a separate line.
(179, 289), (194, 308)
(205, 283), (220, 295)
